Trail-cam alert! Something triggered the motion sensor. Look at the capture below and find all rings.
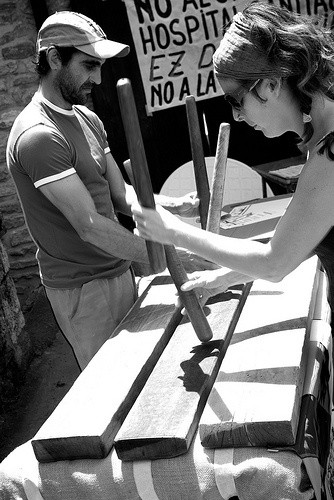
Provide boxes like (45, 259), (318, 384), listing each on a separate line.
(198, 292), (202, 299)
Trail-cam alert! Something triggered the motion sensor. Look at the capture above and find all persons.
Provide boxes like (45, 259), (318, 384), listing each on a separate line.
(6, 10), (223, 372)
(131, 2), (334, 407)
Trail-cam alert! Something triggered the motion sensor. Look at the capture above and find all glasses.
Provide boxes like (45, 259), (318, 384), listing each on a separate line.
(223, 78), (263, 112)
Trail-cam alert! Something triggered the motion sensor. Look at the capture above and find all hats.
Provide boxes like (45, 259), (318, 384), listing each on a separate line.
(34, 9), (132, 59)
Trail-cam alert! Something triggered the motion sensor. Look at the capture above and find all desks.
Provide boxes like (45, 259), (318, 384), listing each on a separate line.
(251, 155), (308, 200)
(0, 255), (334, 500)
